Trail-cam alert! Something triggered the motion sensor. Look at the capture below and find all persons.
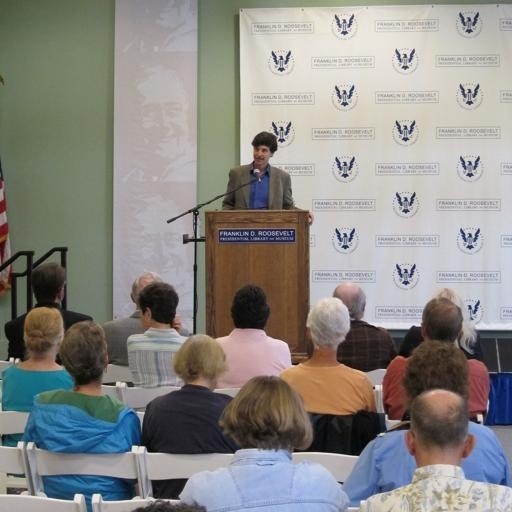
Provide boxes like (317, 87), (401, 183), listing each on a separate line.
(99, 272), (193, 365)
(177, 375), (349, 511)
(221, 130), (314, 229)
(2, 305), (76, 477)
(399, 286), (485, 365)
(126, 282), (189, 389)
(215, 284), (291, 388)
(341, 339), (510, 507)
(4, 261), (94, 367)
(383, 298), (490, 419)
(305, 282), (398, 372)
(141, 331), (242, 499)
(22, 321), (141, 512)
(277, 298), (378, 416)
(358, 387), (511, 512)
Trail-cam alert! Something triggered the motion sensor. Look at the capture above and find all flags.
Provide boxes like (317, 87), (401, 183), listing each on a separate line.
(0, 161), (17, 293)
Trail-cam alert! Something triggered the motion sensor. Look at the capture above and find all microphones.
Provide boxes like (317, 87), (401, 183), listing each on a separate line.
(253, 168), (261, 183)
